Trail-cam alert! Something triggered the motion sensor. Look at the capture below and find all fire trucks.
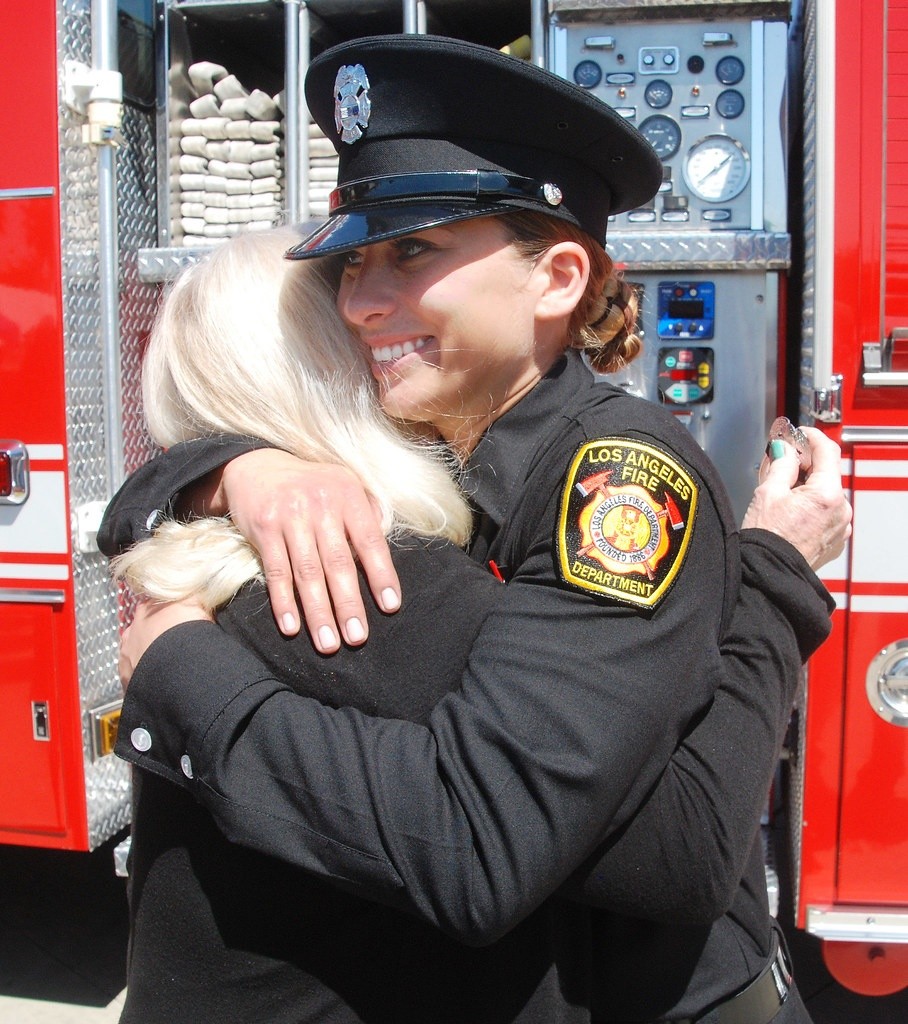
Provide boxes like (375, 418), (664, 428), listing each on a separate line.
(0, 1), (908, 1004)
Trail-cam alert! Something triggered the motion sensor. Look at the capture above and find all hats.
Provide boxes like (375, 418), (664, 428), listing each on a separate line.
(285, 36), (665, 260)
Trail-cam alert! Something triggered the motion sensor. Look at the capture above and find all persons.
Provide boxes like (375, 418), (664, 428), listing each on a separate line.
(99, 29), (856, 1017)
